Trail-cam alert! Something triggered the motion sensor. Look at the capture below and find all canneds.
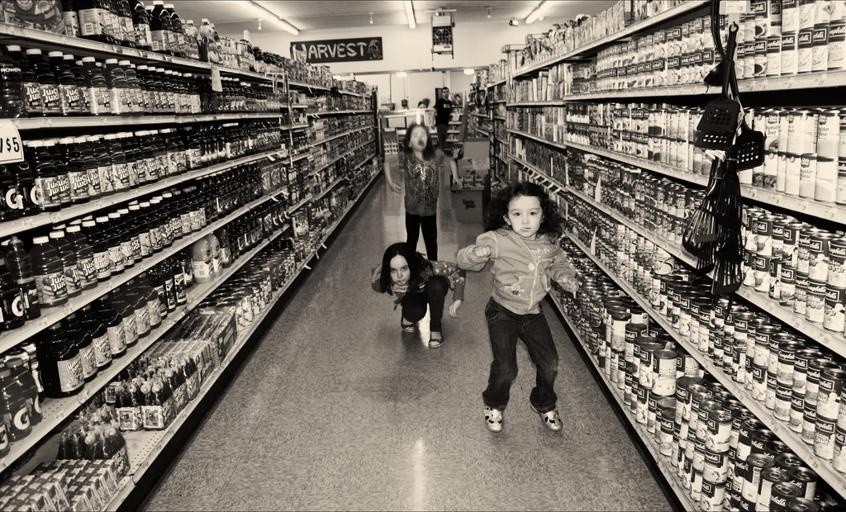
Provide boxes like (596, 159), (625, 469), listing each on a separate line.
(555, 0), (845, 511)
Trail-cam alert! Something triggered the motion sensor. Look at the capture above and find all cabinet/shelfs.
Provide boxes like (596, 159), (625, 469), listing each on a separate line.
(466, 0), (846, 510)
(0, 23), (392, 511)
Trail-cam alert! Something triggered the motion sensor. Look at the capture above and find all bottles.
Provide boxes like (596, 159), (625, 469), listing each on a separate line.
(61, 0), (224, 65)
(279, 126), (308, 154)
(0, 117), (279, 221)
(285, 165), (315, 204)
(0, 361), (196, 475)
(185, 199), (287, 284)
(0, 43), (279, 121)
(0, 221), (187, 361)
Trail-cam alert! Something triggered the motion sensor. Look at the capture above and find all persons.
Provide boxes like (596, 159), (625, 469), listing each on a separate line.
(369, 242), (464, 348)
(401, 89), (463, 147)
(383, 123), (465, 258)
(458, 180), (582, 431)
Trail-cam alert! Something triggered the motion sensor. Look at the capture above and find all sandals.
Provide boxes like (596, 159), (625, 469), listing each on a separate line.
(400, 316), (416, 333)
(429, 334), (444, 348)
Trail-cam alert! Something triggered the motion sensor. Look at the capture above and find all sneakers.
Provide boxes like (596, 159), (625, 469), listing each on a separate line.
(484, 405), (503, 432)
(530, 404), (563, 431)
